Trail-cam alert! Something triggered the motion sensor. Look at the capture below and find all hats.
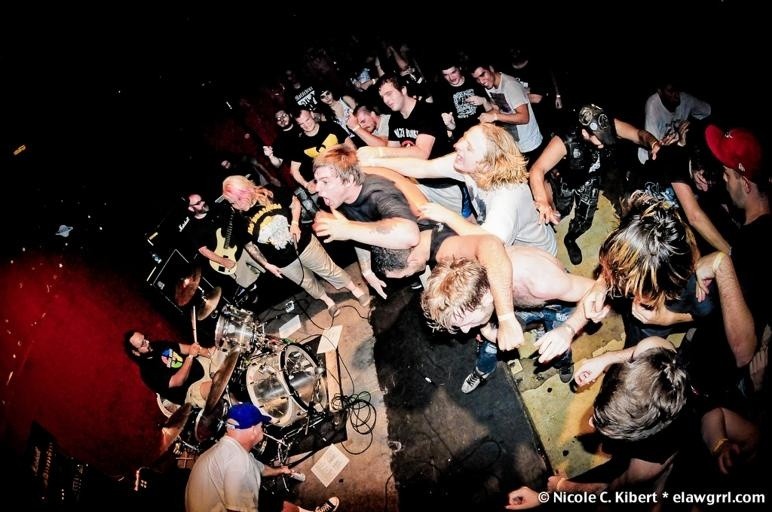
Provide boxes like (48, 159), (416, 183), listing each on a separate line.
(578, 104), (616, 145)
(705, 124), (762, 184)
(227, 404), (271, 428)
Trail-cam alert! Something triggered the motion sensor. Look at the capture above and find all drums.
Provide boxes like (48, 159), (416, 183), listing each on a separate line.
(246, 342), (328, 429)
(215, 305), (254, 351)
(187, 397), (229, 441)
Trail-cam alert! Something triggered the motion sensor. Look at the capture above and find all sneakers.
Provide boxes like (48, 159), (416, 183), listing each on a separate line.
(564, 236), (582, 265)
(461, 366), (496, 394)
(328, 304), (339, 317)
(559, 364), (574, 382)
(358, 294), (370, 306)
(314, 497), (339, 511)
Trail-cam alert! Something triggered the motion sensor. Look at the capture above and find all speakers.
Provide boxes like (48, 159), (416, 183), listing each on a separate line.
(136, 249), (233, 348)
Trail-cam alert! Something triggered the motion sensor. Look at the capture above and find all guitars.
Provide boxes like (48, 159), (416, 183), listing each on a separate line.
(209, 207), (238, 275)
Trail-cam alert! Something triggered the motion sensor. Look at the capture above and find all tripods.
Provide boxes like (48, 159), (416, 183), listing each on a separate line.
(216, 417), (309, 493)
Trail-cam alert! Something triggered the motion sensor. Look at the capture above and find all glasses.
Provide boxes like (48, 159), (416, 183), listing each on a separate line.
(276, 114), (287, 121)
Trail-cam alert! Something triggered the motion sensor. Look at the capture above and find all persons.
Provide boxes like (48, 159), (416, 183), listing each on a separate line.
(123, 41), (770, 510)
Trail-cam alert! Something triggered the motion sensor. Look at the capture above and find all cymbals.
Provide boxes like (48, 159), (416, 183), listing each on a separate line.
(156, 402), (191, 459)
(197, 287), (221, 321)
(176, 260), (201, 306)
(203, 350), (239, 416)
